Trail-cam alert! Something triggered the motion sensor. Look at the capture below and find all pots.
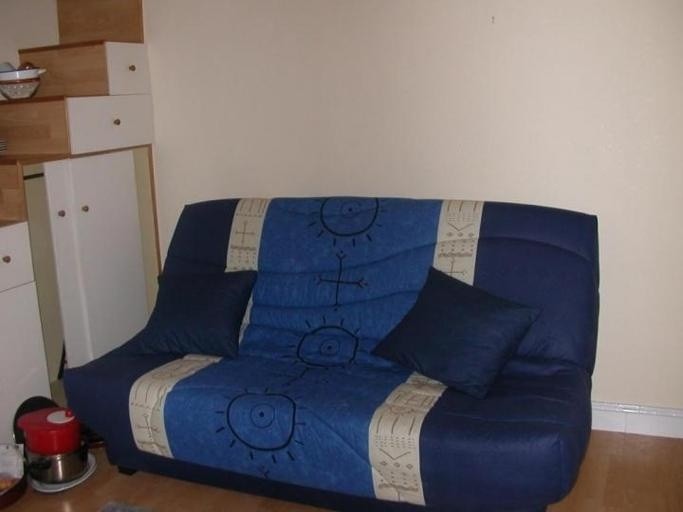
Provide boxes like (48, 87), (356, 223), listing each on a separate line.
(21, 439), (89, 485)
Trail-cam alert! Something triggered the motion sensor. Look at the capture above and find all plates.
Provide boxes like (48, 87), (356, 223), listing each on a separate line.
(28, 453), (98, 494)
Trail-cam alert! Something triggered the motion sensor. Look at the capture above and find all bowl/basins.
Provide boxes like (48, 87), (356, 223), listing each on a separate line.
(0, 62), (41, 101)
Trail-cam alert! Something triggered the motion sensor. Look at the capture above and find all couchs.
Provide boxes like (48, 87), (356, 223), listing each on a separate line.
(60, 194), (602, 512)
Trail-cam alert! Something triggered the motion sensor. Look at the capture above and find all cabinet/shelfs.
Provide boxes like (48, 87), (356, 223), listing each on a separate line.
(107, 40), (152, 96)
(43, 151), (150, 368)
(66, 96), (157, 157)
(0, 221), (52, 447)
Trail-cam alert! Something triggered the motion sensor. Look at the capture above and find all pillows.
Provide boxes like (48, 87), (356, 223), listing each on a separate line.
(370, 265), (545, 403)
(138, 267), (256, 362)
